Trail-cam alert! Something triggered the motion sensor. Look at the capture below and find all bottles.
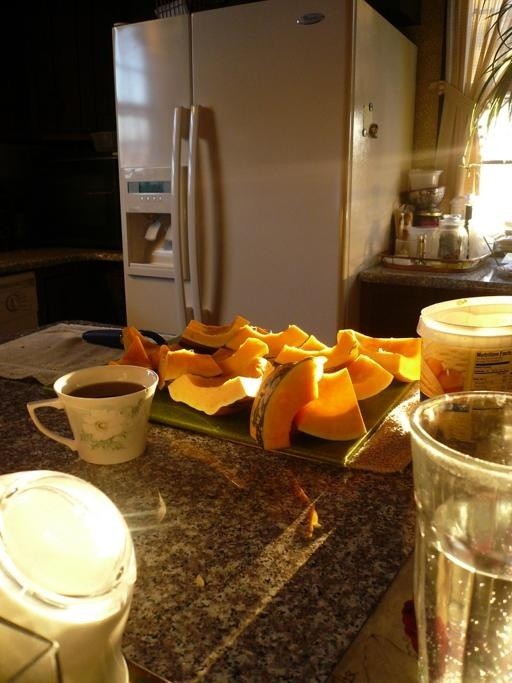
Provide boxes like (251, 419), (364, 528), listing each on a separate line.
(411, 216), (468, 261)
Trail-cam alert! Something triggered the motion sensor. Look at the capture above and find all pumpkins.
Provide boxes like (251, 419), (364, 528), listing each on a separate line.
(102, 312), (422, 451)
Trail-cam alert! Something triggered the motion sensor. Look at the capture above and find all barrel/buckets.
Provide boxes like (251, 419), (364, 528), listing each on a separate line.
(415, 295), (512, 443)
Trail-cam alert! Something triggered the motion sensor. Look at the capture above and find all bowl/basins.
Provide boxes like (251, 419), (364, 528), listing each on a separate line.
(405, 185), (446, 210)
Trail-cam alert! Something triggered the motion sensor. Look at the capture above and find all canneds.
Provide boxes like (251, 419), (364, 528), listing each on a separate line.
(416, 211), (442, 226)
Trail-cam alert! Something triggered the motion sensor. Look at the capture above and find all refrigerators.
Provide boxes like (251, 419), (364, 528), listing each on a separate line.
(112, 0), (418, 349)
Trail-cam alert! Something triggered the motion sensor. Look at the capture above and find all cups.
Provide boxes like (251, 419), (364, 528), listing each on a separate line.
(26, 365), (159, 466)
(410, 390), (512, 683)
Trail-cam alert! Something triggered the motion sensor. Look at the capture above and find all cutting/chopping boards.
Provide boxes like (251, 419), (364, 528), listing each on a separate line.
(105, 332), (418, 467)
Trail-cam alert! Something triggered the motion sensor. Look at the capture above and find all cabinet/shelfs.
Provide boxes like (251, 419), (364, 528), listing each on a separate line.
(359, 280), (464, 337)
(35, 259), (127, 328)
